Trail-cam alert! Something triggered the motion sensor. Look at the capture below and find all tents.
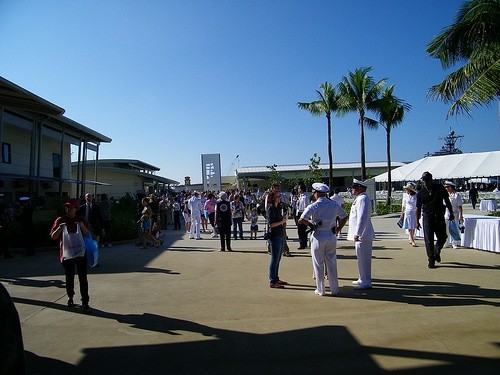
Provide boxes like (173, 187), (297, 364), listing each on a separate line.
(371, 150), (500, 182)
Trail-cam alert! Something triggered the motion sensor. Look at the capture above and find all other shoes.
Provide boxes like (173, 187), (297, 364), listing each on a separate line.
(434, 252), (441, 262)
(67, 298), (74, 306)
(411, 242), (417, 247)
(331, 290), (340, 295)
(269, 284), (285, 289)
(353, 283), (372, 289)
(428, 262), (435, 267)
(452, 245), (459, 249)
(101, 244), (105, 248)
(351, 279), (361, 285)
(277, 280), (288, 285)
(315, 289), (324, 296)
(443, 245), (450, 248)
(81, 302), (90, 311)
(108, 244), (112, 248)
(409, 240), (411, 244)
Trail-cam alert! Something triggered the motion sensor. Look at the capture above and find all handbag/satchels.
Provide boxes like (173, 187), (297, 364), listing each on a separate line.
(84, 231), (98, 269)
(449, 221), (461, 241)
(62, 224), (85, 260)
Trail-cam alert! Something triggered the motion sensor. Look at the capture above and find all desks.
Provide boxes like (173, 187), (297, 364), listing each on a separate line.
(480, 200), (496, 212)
(413, 213), (500, 252)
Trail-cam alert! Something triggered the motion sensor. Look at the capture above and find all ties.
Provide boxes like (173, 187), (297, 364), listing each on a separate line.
(87, 204), (91, 224)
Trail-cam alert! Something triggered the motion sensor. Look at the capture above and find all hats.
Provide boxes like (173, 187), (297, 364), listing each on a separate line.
(444, 181), (456, 188)
(312, 183), (330, 193)
(419, 171), (432, 179)
(348, 179), (368, 190)
(63, 200), (79, 208)
(403, 183), (416, 192)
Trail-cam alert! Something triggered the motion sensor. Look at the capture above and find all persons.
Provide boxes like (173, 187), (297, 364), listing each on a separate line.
(347, 178), (376, 290)
(298, 183), (348, 296)
(80, 182), (345, 251)
(50, 198), (98, 311)
(442, 181), (463, 249)
(416, 171), (455, 268)
(400, 183), (419, 246)
(469, 184), (479, 209)
(265, 190), (287, 289)
